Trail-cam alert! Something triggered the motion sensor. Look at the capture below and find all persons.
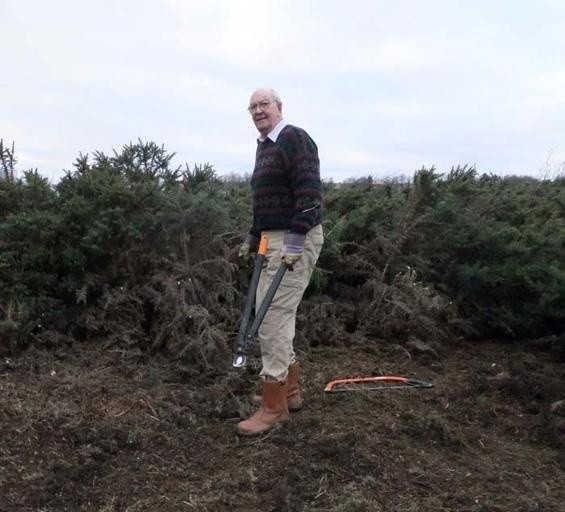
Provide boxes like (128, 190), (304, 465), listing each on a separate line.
(232, 90), (326, 435)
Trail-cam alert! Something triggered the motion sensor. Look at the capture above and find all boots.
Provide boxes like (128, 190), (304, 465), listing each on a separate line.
(236, 375), (290, 437)
(251, 359), (303, 411)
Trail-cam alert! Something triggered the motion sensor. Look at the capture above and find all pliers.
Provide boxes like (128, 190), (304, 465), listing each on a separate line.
(233, 234), (288, 367)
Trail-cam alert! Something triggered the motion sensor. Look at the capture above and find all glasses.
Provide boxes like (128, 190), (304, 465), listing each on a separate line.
(247, 98), (276, 115)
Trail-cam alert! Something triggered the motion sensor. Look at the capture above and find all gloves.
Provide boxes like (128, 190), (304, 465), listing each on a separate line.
(238, 234), (260, 269)
(280, 231), (307, 272)
